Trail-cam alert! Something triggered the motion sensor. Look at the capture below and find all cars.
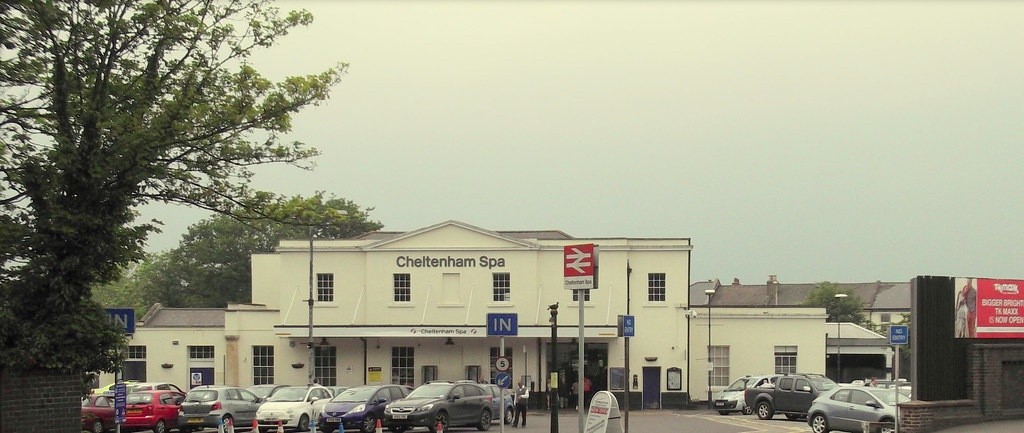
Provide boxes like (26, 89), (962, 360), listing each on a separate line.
(478, 384), (515, 425)
(319, 385), (413, 433)
(806, 386), (911, 433)
(864, 380), (912, 399)
(81, 380), (352, 433)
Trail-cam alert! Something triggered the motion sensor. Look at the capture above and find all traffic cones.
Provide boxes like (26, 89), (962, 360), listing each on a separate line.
(218, 417), (225, 433)
(376, 419), (382, 433)
(252, 420), (259, 433)
(339, 422), (344, 433)
(310, 419), (316, 433)
(437, 422), (443, 433)
(277, 420), (284, 433)
(228, 418), (234, 433)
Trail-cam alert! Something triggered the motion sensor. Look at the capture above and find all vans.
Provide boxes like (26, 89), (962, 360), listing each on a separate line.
(713, 375), (798, 416)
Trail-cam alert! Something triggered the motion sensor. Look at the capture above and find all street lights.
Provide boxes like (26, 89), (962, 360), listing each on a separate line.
(834, 293), (848, 383)
(704, 289), (715, 410)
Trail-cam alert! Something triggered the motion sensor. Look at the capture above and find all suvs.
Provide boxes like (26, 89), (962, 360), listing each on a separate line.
(383, 380), (494, 431)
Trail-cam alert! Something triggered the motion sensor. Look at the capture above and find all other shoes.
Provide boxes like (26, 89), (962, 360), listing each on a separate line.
(511, 425), (517, 427)
(522, 425), (525, 427)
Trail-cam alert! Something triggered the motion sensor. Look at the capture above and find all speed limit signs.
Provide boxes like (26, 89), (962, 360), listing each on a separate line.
(495, 357), (510, 372)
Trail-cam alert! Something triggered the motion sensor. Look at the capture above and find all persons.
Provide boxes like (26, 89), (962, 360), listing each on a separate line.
(546, 375), (592, 410)
(313, 378), (322, 386)
(511, 381), (529, 428)
(955, 278), (977, 339)
(479, 376), (488, 384)
(870, 377), (877, 387)
(761, 380), (768, 386)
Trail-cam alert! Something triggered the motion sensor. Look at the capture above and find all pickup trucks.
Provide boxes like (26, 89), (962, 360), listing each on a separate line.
(744, 374), (839, 420)
